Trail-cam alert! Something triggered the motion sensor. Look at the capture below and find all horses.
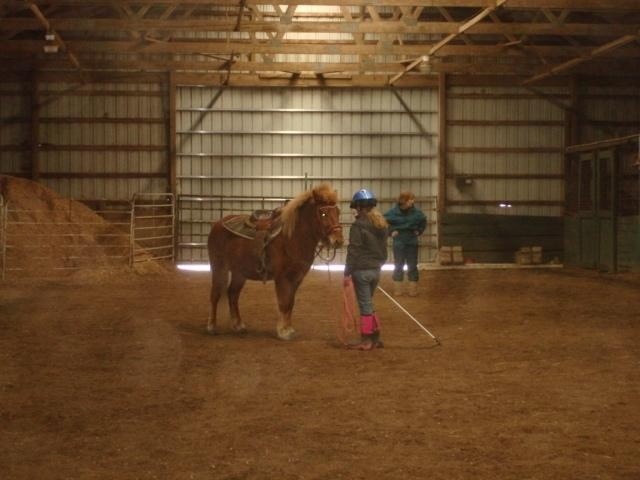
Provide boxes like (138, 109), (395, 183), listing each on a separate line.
(205, 185), (344, 342)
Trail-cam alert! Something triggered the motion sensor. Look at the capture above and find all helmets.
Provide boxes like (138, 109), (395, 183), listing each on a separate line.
(350, 190), (376, 209)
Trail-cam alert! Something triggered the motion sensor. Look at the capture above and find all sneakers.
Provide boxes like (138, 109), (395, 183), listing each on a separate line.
(348, 332), (384, 350)
(392, 280), (403, 295)
(408, 281), (417, 296)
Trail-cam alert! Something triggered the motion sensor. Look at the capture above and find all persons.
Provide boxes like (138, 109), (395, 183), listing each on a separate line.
(382, 191), (427, 297)
(343, 188), (388, 351)
